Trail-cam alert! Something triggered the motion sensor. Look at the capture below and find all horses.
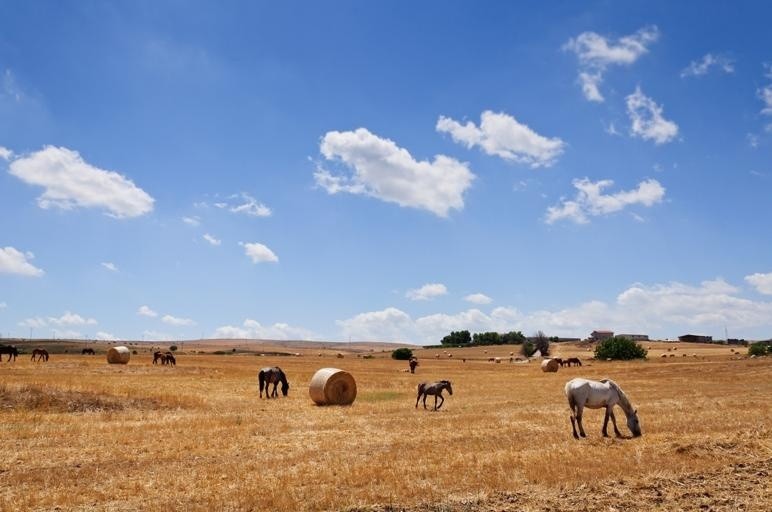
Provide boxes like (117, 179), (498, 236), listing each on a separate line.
(81, 348), (95, 355)
(409, 355), (419, 373)
(554, 358), (582, 368)
(0, 344), (18, 362)
(259, 367), (289, 399)
(153, 351), (176, 368)
(31, 349), (49, 364)
(565, 379), (641, 440)
(416, 381), (452, 411)
